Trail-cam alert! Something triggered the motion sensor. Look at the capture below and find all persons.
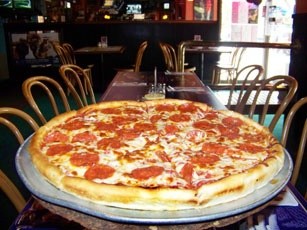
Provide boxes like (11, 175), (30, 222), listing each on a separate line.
(26, 31), (38, 59)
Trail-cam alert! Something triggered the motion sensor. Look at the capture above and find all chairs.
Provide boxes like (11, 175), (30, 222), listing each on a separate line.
(160, 43), (197, 74)
(248, 75), (298, 133)
(63, 42), (92, 95)
(216, 48), (246, 85)
(55, 46), (93, 95)
(227, 65), (264, 111)
(59, 65), (96, 107)
(280, 97), (307, 200)
(212, 46), (246, 85)
(0, 107), (40, 214)
(22, 76), (71, 125)
(116, 40), (147, 74)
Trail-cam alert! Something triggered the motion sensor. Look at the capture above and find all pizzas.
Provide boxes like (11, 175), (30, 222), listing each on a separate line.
(27, 99), (285, 211)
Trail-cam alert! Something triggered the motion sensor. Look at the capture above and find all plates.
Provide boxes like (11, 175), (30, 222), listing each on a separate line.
(15, 131), (293, 224)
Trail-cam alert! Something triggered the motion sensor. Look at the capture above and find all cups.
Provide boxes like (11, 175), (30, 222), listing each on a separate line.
(149, 83), (165, 99)
